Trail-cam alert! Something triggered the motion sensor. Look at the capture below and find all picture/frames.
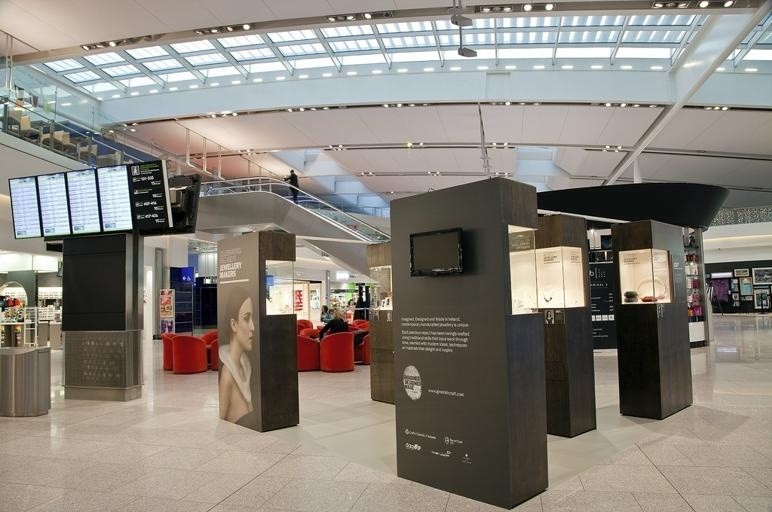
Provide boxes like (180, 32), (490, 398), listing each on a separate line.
(733, 268), (772, 310)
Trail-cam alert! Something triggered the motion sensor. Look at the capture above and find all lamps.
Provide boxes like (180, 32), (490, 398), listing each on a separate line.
(450, 0), (477, 58)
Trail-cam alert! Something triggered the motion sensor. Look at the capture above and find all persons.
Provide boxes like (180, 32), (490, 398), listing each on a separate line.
(320, 304), (333, 323)
(218, 287), (258, 430)
(315, 307), (350, 343)
(285, 168), (300, 204)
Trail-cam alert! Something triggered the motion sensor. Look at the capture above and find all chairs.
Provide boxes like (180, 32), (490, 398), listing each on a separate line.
(0, 110), (122, 169)
(159, 319), (369, 375)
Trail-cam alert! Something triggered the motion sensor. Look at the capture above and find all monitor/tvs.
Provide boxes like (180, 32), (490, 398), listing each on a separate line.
(408, 228), (465, 277)
(140, 173), (203, 238)
